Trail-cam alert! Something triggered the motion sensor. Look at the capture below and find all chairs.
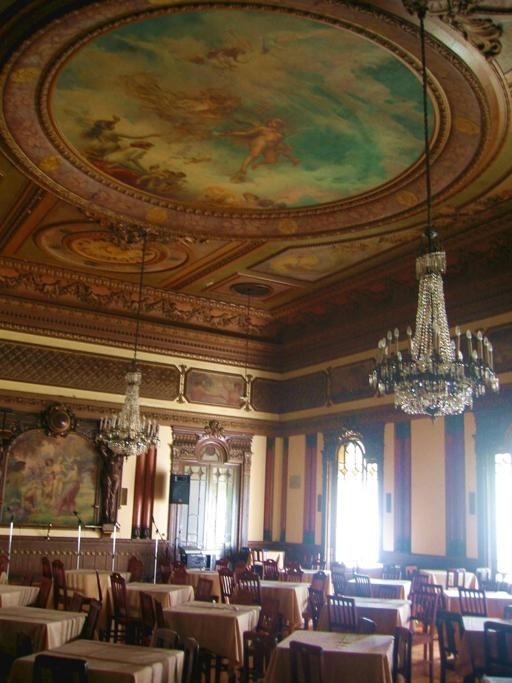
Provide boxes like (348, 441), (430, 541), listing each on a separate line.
(0, 547), (512, 683)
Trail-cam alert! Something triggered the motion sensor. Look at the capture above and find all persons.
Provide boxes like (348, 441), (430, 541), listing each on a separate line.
(99, 444), (122, 523)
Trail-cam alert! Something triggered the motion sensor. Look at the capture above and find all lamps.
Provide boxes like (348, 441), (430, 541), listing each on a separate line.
(94, 232), (160, 459)
(368, 0), (500, 422)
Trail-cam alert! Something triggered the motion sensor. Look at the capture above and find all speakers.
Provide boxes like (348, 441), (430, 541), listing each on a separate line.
(169, 474), (191, 503)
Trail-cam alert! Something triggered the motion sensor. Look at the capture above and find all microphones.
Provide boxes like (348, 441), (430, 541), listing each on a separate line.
(74, 511), (81, 517)
(46, 522), (53, 540)
